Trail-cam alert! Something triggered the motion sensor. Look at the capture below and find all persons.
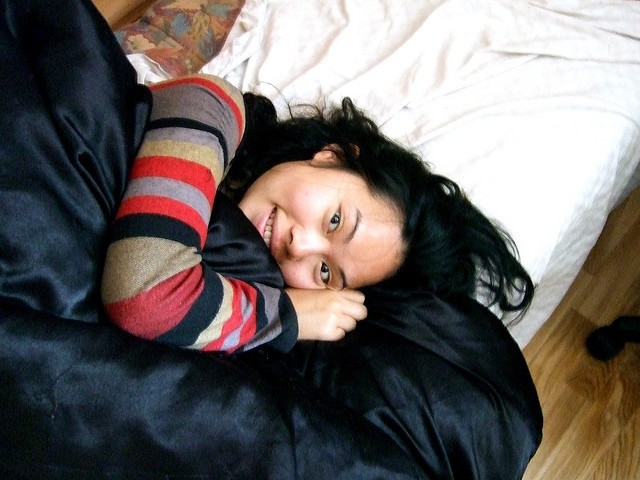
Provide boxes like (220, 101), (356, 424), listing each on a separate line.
(98, 74), (536, 357)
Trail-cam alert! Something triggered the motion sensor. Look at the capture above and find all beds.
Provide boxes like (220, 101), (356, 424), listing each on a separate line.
(0, 0), (640, 478)
